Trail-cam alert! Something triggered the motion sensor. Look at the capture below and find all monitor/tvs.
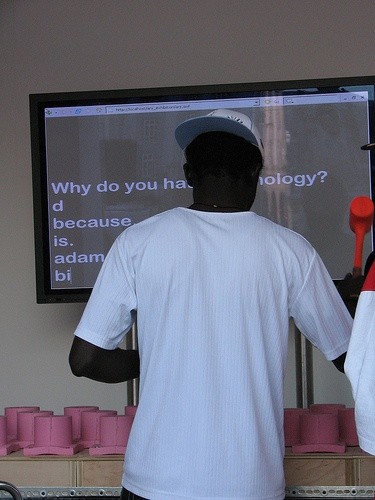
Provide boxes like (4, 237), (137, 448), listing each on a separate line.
(29, 75), (375, 304)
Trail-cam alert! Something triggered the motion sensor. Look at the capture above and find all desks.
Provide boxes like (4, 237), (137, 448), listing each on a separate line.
(0, 453), (375, 500)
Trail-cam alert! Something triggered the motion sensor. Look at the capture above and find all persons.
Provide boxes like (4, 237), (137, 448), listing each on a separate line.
(69, 109), (354, 500)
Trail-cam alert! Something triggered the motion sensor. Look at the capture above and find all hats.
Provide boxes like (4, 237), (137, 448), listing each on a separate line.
(176, 109), (266, 153)
